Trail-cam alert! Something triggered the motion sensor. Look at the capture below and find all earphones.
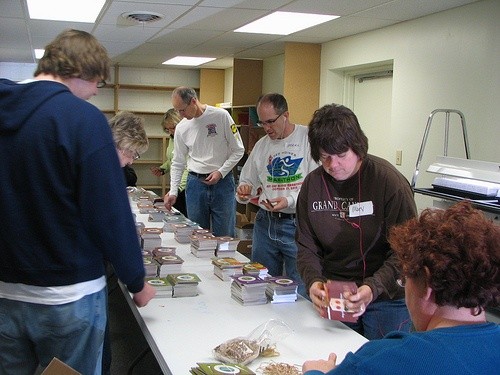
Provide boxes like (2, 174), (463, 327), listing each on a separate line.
(283, 116), (287, 120)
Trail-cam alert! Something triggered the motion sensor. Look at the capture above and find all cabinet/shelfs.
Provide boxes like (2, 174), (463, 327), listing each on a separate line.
(87, 83), (266, 228)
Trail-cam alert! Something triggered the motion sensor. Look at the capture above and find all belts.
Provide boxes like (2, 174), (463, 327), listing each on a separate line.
(189, 171), (211, 179)
(266, 210), (296, 219)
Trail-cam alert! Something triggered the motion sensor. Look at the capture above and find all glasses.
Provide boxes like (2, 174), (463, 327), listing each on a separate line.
(256, 113), (282, 128)
(397, 275), (410, 288)
(134, 150), (140, 160)
(164, 126), (176, 132)
(174, 100), (192, 114)
(97, 79), (106, 88)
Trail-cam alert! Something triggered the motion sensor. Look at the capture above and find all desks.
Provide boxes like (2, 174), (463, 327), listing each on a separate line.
(108, 188), (370, 375)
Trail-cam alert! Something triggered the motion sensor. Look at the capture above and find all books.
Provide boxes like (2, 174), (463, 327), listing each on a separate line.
(132, 213), (184, 278)
(172, 221), (241, 259)
(145, 273), (202, 298)
(132, 190), (186, 232)
(188, 362), (256, 375)
(211, 256), (298, 305)
(320, 280), (358, 323)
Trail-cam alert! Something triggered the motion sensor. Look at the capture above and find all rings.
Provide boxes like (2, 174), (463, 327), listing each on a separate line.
(360, 306), (365, 311)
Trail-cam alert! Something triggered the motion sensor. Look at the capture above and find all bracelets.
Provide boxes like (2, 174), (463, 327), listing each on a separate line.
(285, 196), (293, 208)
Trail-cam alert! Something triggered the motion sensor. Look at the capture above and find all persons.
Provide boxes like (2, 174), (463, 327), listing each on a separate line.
(295, 104), (419, 341)
(235, 92), (323, 302)
(0, 28), (156, 375)
(164, 86), (245, 237)
(107, 111), (149, 168)
(151, 108), (188, 218)
(302, 200), (500, 375)
